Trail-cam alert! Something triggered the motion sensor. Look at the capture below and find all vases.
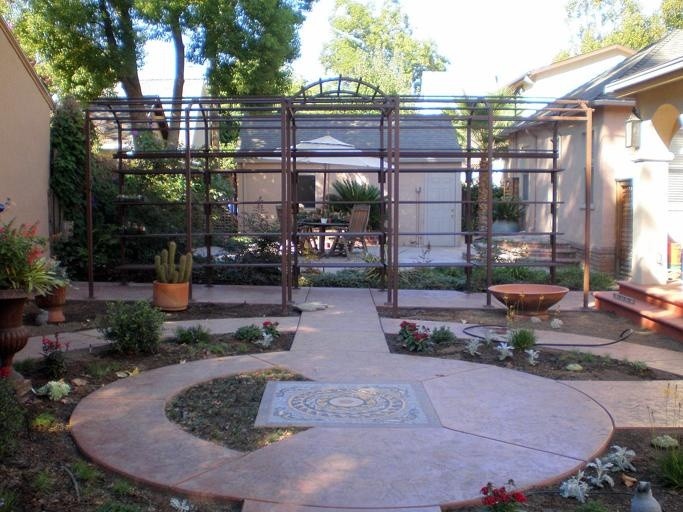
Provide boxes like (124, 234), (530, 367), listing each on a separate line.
(0, 289), (33, 358)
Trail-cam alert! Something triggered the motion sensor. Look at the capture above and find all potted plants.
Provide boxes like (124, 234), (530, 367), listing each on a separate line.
(152, 241), (193, 312)
(35, 256), (70, 323)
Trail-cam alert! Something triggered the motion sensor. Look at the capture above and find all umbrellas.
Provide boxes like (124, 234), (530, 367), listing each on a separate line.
(258, 134), (395, 218)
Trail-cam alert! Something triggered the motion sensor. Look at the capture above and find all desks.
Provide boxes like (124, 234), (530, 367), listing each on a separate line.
(303, 223), (350, 252)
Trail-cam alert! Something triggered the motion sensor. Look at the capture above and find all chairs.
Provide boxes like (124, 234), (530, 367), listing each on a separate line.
(328, 204), (371, 257)
(275, 205), (317, 255)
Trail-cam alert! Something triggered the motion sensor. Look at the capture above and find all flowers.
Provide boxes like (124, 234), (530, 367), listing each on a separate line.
(0, 198), (73, 297)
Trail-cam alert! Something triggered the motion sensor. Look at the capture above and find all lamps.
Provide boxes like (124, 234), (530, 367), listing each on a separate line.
(623, 111), (642, 149)
